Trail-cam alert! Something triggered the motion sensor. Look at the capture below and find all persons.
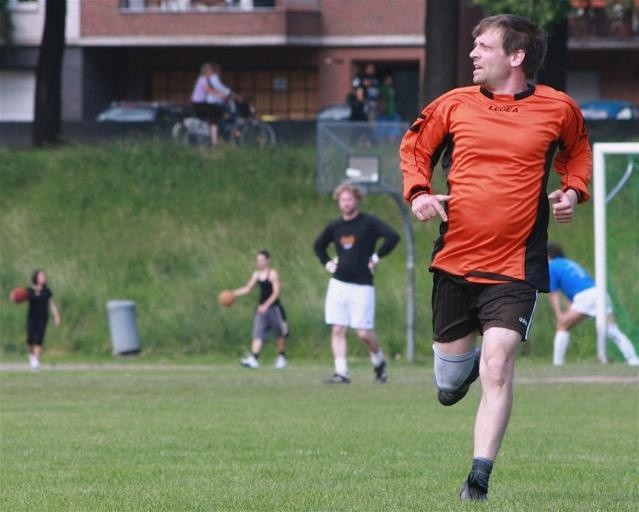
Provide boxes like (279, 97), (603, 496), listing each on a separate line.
(542, 237), (639, 366)
(9, 265), (60, 369)
(345, 79), (379, 145)
(311, 183), (399, 386)
(206, 61), (244, 106)
(231, 250), (291, 369)
(185, 63), (224, 147)
(394, 11), (593, 501)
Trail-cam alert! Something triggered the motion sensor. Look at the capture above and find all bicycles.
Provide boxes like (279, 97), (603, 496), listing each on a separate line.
(172, 114), (276, 148)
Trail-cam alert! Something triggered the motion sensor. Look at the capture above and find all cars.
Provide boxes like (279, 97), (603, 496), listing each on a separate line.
(92, 101), (182, 140)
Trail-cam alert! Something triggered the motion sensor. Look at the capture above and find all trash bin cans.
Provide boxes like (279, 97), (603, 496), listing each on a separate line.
(104, 300), (143, 356)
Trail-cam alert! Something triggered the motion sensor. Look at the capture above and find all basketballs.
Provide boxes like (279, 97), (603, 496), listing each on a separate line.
(9, 285), (30, 304)
(218, 288), (236, 308)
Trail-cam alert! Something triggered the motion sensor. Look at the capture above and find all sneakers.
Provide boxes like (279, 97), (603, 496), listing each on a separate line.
(274, 354), (287, 371)
(239, 354), (260, 368)
(323, 374), (350, 384)
(373, 360), (387, 384)
(438, 351), (481, 406)
(459, 475), (488, 503)
